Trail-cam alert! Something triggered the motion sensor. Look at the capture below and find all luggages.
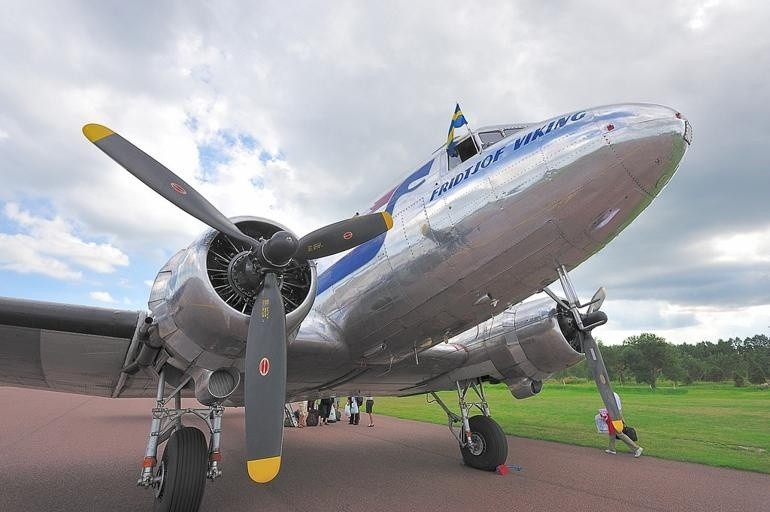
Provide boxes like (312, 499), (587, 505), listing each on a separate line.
(298, 412), (304, 428)
(307, 410), (319, 426)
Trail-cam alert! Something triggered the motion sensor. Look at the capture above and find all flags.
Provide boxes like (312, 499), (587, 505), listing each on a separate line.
(446, 104), (467, 158)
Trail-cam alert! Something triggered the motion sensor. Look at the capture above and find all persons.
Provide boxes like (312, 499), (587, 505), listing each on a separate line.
(291, 396), (363, 426)
(605, 391), (644, 458)
(365, 396), (375, 427)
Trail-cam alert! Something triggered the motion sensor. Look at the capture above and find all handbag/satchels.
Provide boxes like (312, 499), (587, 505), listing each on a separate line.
(345, 404), (351, 417)
(616, 426), (637, 441)
(351, 400), (359, 414)
(596, 415), (609, 433)
(329, 406), (336, 421)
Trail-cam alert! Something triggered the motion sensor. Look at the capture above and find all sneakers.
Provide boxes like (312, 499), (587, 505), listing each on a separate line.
(320, 422), (329, 426)
(634, 447), (643, 458)
(348, 422), (358, 425)
(368, 424), (374, 427)
(605, 449), (616, 455)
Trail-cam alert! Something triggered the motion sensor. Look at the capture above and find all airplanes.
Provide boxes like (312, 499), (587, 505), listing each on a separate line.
(1, 102), (693, 510)
